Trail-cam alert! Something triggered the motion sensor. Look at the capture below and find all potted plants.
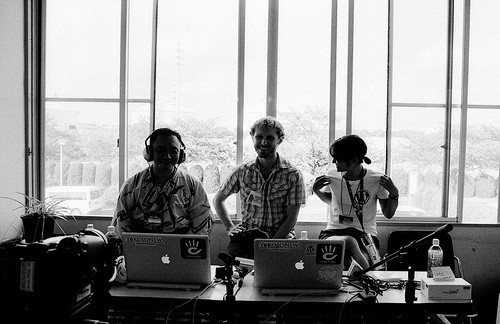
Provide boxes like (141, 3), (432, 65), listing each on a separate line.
(6, 192), (45, 243)
(44, 196), (66, 239)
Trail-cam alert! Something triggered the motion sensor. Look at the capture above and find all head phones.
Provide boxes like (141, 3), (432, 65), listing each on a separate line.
(143, 128), (186, 164)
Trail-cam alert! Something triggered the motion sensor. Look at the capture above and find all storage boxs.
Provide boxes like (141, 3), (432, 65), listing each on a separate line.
(421, 278), (472, 301)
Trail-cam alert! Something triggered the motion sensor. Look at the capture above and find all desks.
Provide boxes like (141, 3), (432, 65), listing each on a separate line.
(110, 264), (472, 324)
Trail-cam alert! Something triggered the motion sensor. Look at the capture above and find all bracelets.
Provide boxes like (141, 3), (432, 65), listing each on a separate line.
(389, 194), (399, 200)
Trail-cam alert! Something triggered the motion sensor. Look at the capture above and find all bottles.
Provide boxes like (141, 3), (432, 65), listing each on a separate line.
(426, 239), (443, 279)
(86, 224), (93, 228)
(299, 231), (308, 239)
(105, 225), (118, 237)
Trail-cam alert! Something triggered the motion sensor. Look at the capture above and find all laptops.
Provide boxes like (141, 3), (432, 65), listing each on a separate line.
(253, 238), (346, 295)
(122, 232), (216, 290)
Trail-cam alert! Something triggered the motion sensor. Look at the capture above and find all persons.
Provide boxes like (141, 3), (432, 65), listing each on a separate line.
(248, 194), (253, 203)
(213, 116), (306, 258)
(111, 128), (215, 284)
(313, 135), (398, 271)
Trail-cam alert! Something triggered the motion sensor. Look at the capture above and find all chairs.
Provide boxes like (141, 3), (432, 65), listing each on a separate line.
(385, 230), (456, 275)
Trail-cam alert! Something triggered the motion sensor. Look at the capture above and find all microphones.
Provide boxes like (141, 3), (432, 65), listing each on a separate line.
(218, 252), (240, 266)
